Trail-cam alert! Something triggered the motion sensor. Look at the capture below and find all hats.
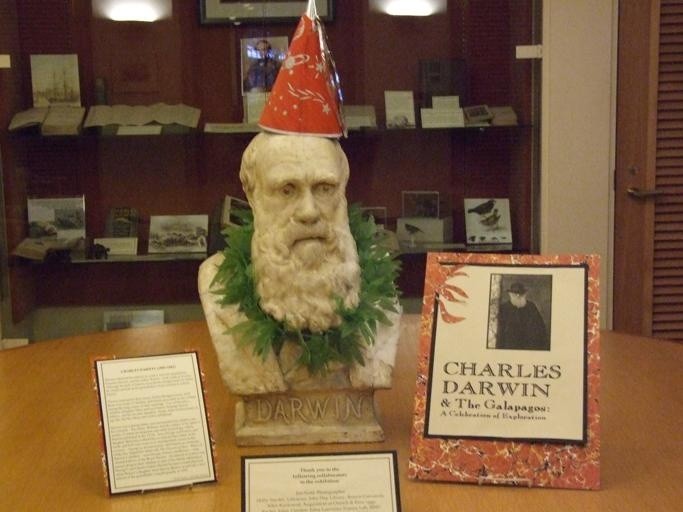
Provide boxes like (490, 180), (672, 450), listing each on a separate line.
(506, 283), (530, 294)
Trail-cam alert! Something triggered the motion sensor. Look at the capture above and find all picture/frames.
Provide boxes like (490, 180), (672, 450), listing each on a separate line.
(404, 246), (606, 489)
(192, 1), (338, 31)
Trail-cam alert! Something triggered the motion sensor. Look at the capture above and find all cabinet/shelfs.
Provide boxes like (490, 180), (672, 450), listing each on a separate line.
(0, 0), (544, 325)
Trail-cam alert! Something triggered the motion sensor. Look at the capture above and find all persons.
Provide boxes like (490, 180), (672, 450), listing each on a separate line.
(497, 284), (548, 349)
(243, 40), (279, 92)
(197, 133), (401, 394)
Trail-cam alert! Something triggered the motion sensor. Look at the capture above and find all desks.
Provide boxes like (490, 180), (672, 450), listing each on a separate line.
(0, 320), (681, 512)
